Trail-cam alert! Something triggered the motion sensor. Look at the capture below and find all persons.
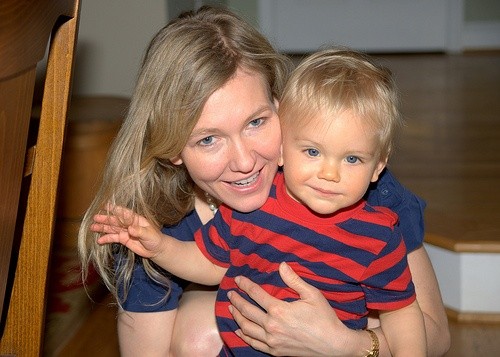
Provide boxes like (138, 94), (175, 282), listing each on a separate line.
(76, 6), (452, 357)
(90, 48), (427, 357)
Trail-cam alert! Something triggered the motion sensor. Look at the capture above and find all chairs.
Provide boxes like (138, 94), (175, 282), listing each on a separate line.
(0, 0), (82, 357)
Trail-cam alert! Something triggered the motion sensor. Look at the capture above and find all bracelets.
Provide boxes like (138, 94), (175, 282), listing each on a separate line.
(364, 329), (380, 357)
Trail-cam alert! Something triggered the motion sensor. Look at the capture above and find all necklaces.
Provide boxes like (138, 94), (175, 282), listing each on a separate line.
(203, 191), (218, 216)
